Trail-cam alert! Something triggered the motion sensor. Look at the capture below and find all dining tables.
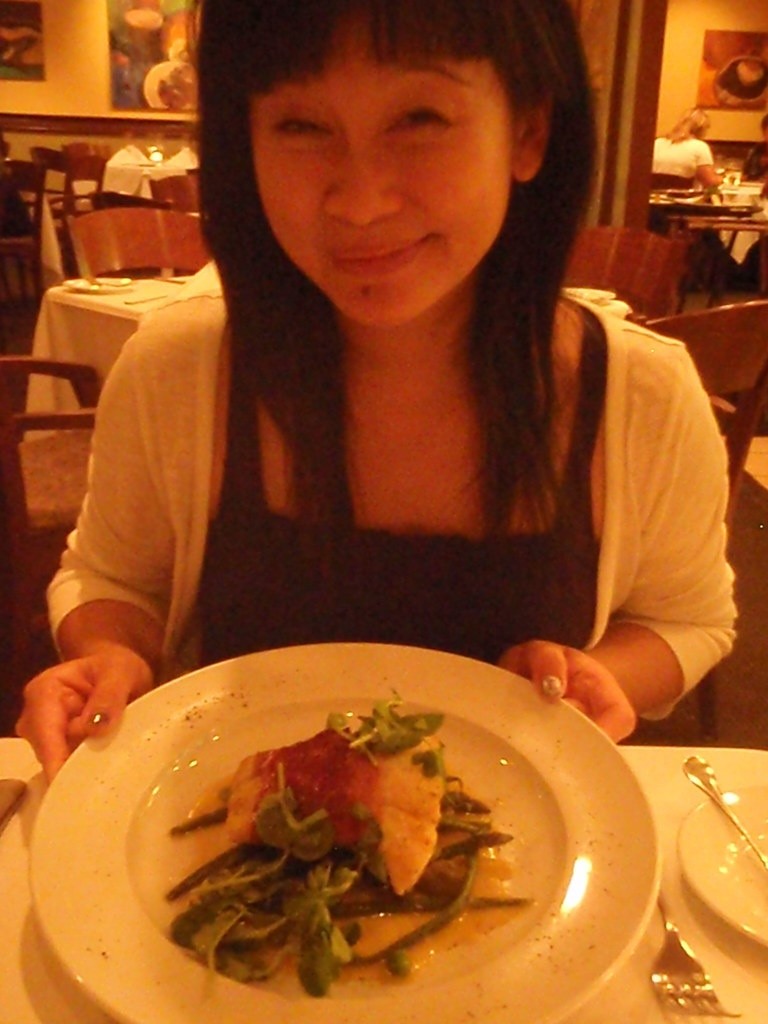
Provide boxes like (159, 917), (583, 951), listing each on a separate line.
(23, 272), (193, 444)
(0, 736), (768, 1023)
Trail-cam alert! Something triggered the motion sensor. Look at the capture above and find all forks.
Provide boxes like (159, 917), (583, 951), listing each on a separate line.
(651, 890), (741, 1019)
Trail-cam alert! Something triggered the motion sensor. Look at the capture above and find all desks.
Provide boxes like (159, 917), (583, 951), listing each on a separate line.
(663, 212), (768, 308)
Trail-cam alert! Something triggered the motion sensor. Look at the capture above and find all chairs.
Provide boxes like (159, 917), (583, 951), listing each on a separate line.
(2, 144), (767, 753)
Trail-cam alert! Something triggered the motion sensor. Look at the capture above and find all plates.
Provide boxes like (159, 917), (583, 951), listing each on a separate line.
(31, 643), (666, 1024)
(678, 785), (767, 947)
(63, 278), (132, 294)
(566, 289), (617, 303)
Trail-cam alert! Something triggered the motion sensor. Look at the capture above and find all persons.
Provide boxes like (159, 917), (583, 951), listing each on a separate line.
(740, 115), (768, 182)
(652, 108), (724, 190)
(13, 0), (739, 796)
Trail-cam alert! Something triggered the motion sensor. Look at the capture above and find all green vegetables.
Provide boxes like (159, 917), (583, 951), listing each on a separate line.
(166, 689), (535, 1001)
